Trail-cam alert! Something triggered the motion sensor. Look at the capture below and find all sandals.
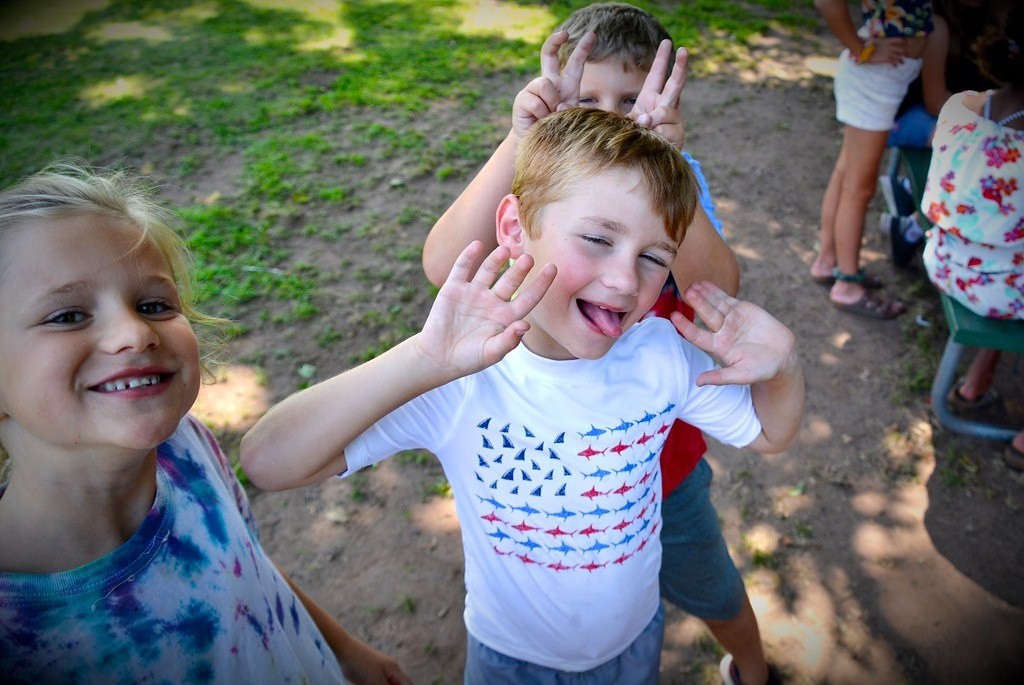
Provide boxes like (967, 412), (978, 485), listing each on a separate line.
(812, 266), (906, 319)
(949, 384), (999, 409)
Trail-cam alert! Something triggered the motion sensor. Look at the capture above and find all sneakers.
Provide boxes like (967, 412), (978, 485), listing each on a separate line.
(718, 653), (742, 682)
(877, 172), (919, 270)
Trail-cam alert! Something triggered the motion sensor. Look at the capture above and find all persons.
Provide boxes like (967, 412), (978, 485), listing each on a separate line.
(920, 78), (1024, 465)
(420, 4), (800, 684)
(808, 0), (951, 320)
(237, 106), (806, 685)
(1, 157), (412, 685)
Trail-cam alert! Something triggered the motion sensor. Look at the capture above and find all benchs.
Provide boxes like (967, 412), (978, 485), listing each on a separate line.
(880, 96), (1024, 440)
